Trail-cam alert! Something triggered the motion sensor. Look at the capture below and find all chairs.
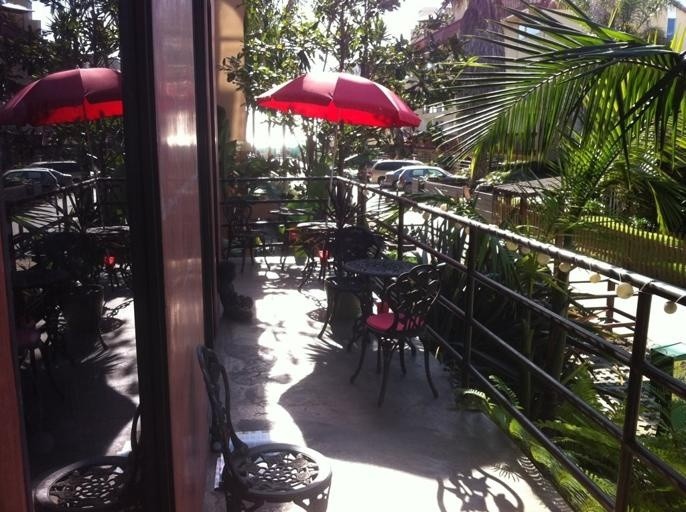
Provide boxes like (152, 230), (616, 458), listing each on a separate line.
(197, 341), (334, 512)
(350, 262), (442, 406)
(227, 203), (425, 352)
(27, 395), (158, 511)
(11, 205), (132, 394)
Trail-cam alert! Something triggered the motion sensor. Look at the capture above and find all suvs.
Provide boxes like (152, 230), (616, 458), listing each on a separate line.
(396, 167), (469, 195)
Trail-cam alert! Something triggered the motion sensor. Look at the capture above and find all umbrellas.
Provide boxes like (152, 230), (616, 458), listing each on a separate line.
(254, 72), (422, 224)
(1, 63), (124, 227)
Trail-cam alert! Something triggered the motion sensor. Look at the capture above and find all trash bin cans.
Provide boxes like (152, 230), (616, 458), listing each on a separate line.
(649, 342), (686, 432)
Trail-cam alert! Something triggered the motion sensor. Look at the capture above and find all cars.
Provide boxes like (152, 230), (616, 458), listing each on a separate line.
(340, 158), (425, 192)
(1, 161), (101, 208)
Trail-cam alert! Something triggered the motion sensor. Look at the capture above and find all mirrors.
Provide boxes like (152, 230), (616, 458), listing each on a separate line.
(0, 0), (178, 511)
